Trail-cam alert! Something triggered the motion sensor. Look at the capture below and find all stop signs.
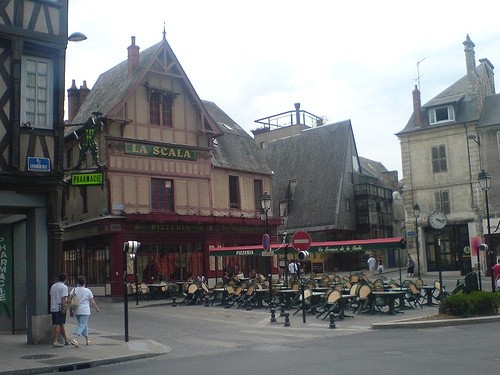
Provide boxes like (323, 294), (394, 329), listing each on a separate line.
(290, 231), (312, 251)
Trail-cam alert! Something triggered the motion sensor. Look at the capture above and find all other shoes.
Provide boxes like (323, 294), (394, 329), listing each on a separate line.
(72, 339), (79, 348)
(53, 343), (64, 348)
(86, 341), (91, 346)
(65, 339), (71, 345)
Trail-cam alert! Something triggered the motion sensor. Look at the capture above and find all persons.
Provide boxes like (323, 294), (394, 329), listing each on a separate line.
(69, 275), (99, 348)
(188, 273), (194, 286)
(377, 256), (384, 273)
(494, 259), (500, 290)
(288, 260), (297, 280)
(196, 273), (206, 287)
(49, 274), (71, 348)
(221, 269), (266, 288)
(367, 255), (376, 272)
(407, 253), (415, 277)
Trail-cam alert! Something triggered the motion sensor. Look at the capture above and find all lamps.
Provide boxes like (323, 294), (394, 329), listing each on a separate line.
(468, 134), (480, 145)
(393, 191), (403, 201)
(69, 32), (87, 41)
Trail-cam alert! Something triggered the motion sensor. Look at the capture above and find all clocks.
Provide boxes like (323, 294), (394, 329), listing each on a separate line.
(429, 210), (446, 229)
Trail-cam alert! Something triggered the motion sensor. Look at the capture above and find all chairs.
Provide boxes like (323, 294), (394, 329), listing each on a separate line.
(133, 274), (449, 319)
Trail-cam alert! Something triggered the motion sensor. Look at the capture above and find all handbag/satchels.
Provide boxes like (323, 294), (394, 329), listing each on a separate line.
(69, 294), (78, 311)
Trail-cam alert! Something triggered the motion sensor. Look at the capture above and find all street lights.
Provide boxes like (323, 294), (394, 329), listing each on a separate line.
(260, 191), (277, 322)
(477, 168), (496, 292)
(413, 202), (421, 279)
